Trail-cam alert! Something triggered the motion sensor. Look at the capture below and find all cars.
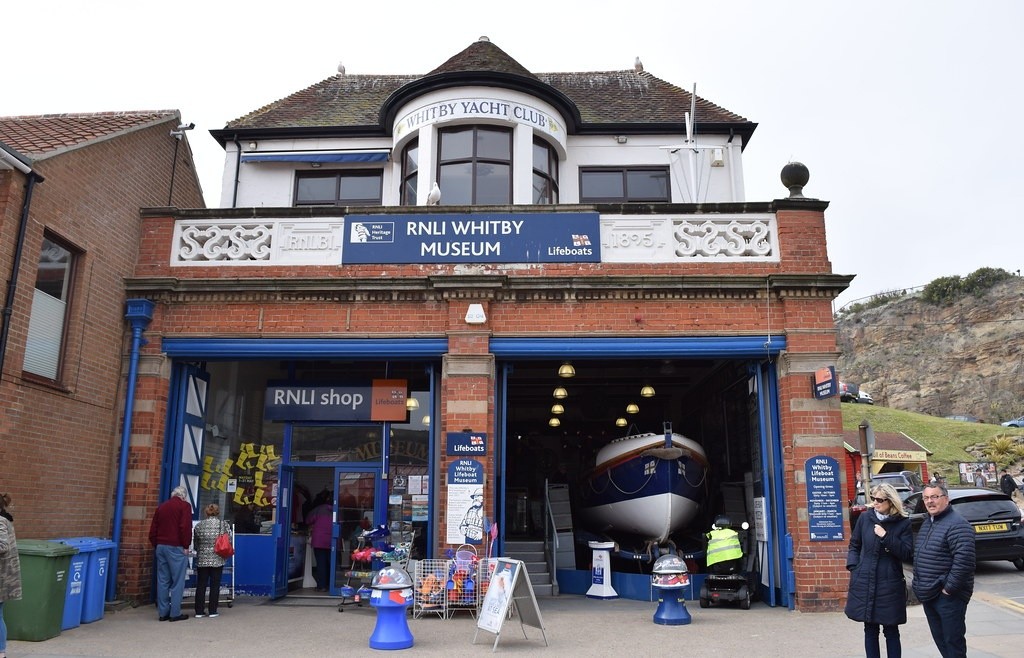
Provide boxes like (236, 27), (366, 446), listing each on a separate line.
(1001, 415), (1024, 428)
(943, 415), (980, 423)
(901, 487), (1024, 572)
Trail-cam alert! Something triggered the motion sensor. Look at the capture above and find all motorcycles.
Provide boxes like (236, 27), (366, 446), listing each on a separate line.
(700, 522), (751, 610)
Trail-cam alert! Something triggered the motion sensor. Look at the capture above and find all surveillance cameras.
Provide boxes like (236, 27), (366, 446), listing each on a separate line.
(177, 123), (195, 131)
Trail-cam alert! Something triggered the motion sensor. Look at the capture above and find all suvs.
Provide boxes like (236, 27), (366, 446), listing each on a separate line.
(850, 471), (928, 532)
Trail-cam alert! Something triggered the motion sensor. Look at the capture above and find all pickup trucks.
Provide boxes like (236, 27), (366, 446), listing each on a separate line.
(851, 390), (874, 404)
(839, 381), (859, 402)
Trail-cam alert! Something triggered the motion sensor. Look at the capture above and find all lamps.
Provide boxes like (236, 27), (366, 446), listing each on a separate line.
(552, 386), (568, 399)
(405, 397), (418, 410)
(551, 404), (564, 414)
(249, 141), (257, 149)
(311, 162), (321, 168)
(558, 360), (575, 377)
(422, 415), (430, 426)
(616, 418), (627, 427)
(618, 135), (627, 143)
(549, 417), (560, 426)
(640, 384), (655, 397)
(625, 401), (639, 413)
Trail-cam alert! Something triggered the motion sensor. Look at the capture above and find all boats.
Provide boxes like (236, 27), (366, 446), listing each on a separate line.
(575, 420), (711, 545)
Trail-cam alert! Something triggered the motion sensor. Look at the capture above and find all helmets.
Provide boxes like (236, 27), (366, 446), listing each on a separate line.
(713, 517), (732, 526)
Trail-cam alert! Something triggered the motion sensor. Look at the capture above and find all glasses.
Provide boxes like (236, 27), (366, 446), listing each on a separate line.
(870, 496), (888, 503)
(921, 494), (945, 500)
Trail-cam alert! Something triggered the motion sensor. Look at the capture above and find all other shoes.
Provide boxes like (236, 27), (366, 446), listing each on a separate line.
(314, 588), (319, 590)
(159, 615), (168, 620)
(319, 587), (327, 591)
(169, 614), (189, 622)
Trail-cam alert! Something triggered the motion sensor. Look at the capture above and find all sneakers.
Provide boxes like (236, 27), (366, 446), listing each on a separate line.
(208, 610), (219, 617)
(194, 611), (206, 617)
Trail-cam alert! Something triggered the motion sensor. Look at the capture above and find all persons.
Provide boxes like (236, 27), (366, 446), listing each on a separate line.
(150, 487), (193, 621)
(913, 483), (976, 658)
(1000, 469), (1019, 500)
(974, 468), (988, 488)
(193, 503), (233, 617)
(294, 486), (372, 591)
(0, 494), (22, 658)
(844, 482), (914, 658)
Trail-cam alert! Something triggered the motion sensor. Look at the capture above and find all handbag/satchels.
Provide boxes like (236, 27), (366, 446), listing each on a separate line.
(215, 520), (233, 558)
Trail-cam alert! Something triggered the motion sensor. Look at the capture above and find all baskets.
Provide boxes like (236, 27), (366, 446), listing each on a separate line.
(455, 544), (477, 570)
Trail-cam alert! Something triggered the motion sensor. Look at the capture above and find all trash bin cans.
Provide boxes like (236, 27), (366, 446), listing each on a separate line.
(71, 537), (118, 624)
(5, 538), (80, 642)
(47, 538), (96, 631)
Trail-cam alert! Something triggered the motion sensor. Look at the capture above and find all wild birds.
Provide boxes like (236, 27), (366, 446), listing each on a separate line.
(426, 182), (442, 205)
